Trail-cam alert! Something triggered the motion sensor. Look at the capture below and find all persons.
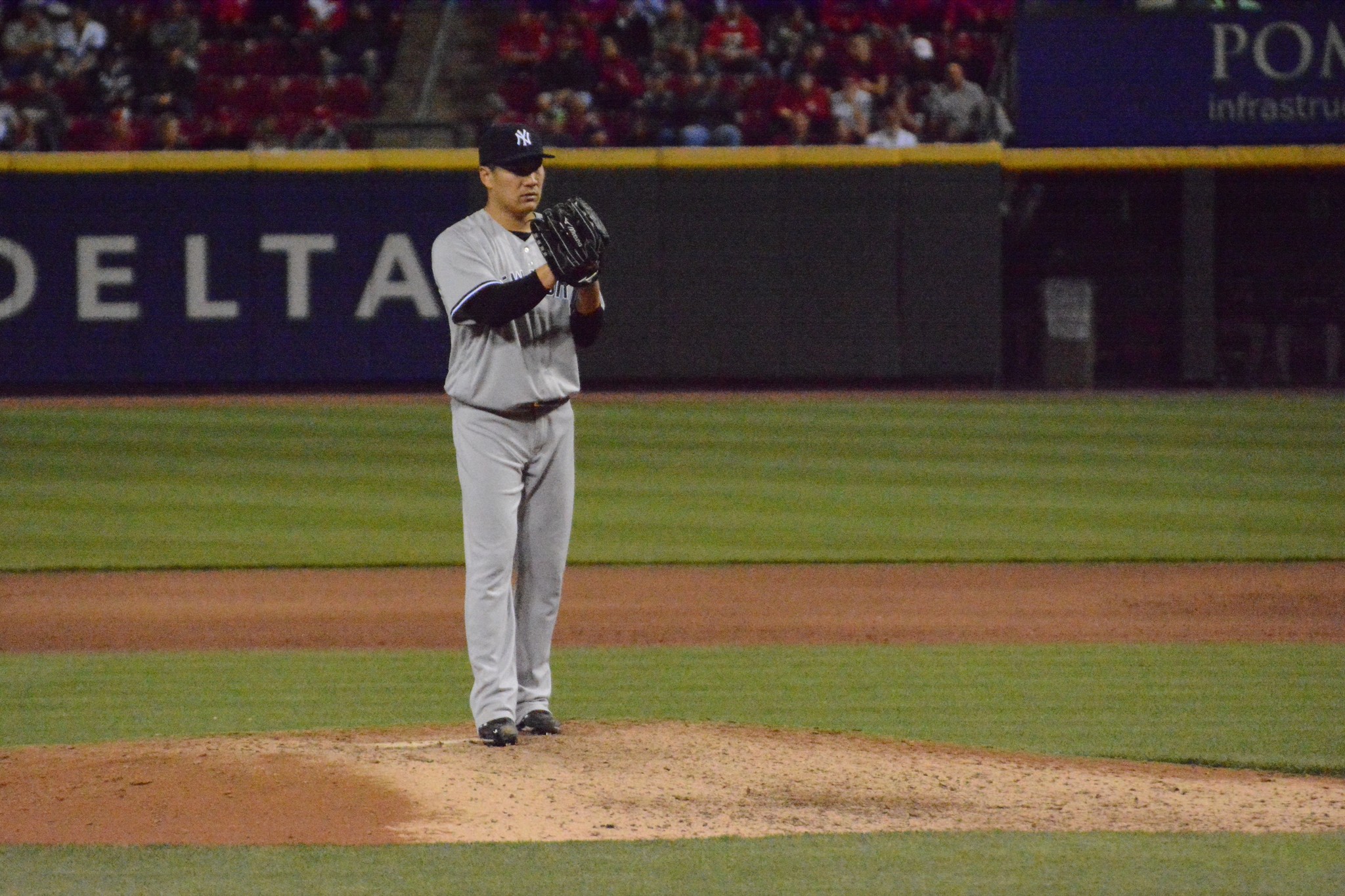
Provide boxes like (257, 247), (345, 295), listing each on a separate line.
(432, 123), (606, 749)
(488, 0), (1015, 147)
(0, 0), (392, 149)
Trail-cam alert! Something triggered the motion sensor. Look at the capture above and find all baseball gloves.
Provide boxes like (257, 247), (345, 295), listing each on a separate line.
(528, 195), (612, 287)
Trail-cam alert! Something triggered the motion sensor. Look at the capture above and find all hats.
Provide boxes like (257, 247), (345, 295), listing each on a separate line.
(479, 123), (555, 165)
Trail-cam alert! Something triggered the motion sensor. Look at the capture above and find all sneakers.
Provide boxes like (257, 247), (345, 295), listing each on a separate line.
(516, 710), (560, 734)
(478, 718), (518, 746)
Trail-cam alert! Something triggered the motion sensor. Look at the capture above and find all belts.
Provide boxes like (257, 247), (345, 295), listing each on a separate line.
(486, 397), (569, 419)
